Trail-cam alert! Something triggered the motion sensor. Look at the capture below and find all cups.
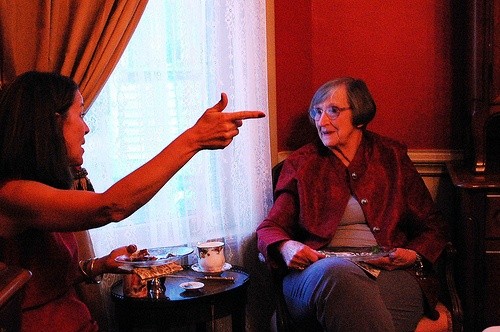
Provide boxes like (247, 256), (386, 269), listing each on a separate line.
(198, 242), (225, 271)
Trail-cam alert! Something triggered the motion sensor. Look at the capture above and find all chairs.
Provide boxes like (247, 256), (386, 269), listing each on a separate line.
(264, 158), (465, 332)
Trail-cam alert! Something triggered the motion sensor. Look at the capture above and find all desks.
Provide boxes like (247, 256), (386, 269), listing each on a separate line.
(112, 265), (249, 332)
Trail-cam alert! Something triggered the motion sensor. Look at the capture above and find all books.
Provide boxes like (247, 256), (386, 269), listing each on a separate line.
(314, 249), (397, 278)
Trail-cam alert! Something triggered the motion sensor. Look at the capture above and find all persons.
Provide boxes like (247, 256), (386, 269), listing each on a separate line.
(0, 72), (266, 332)
(256, 78), (444, 332)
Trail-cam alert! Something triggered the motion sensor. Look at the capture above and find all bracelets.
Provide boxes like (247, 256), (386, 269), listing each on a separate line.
(78, 257), (102, 285)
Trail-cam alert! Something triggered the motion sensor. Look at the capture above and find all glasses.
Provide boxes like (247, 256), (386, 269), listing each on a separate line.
(308, 105), (351, 119)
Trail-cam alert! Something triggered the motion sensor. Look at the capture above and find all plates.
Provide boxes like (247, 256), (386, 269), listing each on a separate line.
(114, 246), (194, 267)
(179, 282), (205, 290)
(191, 263), (232, 276)
(316, 252), (394, 261)
